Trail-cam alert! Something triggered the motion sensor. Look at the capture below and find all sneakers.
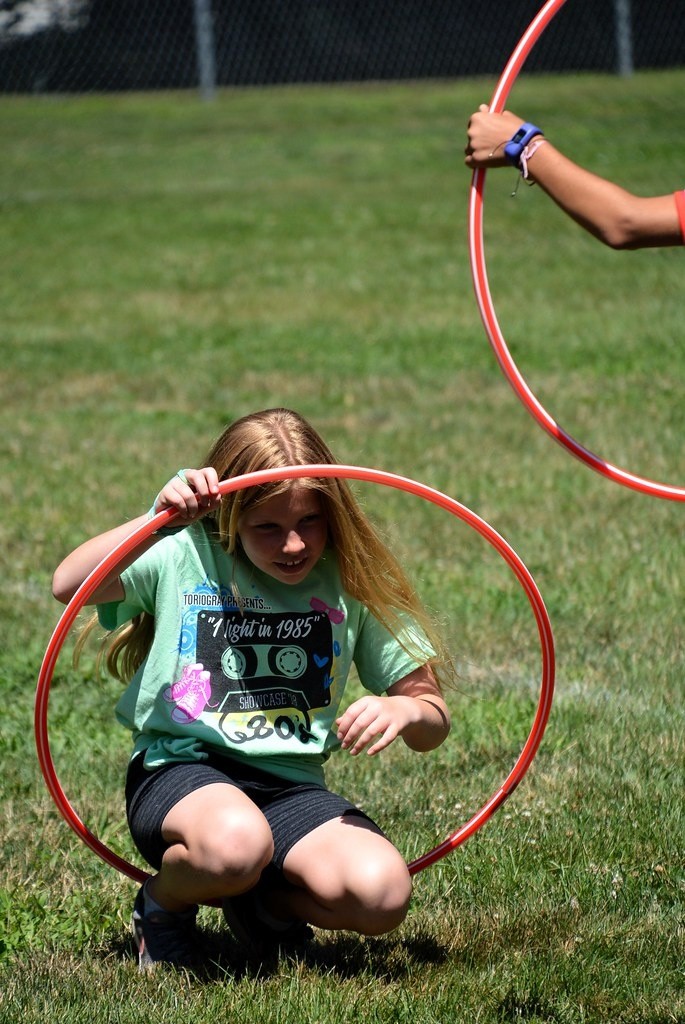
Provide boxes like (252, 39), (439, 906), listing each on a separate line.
(220, 893), (338, 981)
(129, 885), (219, 985)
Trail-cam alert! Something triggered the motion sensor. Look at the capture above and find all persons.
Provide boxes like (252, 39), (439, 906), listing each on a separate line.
(50, 409), (451, 959)
(464, 102), (685, 251)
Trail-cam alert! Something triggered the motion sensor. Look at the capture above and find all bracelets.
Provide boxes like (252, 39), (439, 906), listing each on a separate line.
(488, 135), (551, 197)
(145, 474), (190, 537)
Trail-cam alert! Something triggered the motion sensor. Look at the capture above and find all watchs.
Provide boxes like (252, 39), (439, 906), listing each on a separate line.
(503, 123), (545, 171)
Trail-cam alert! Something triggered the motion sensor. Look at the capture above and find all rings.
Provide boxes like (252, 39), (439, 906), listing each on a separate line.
(175, 468), (193, 486)
